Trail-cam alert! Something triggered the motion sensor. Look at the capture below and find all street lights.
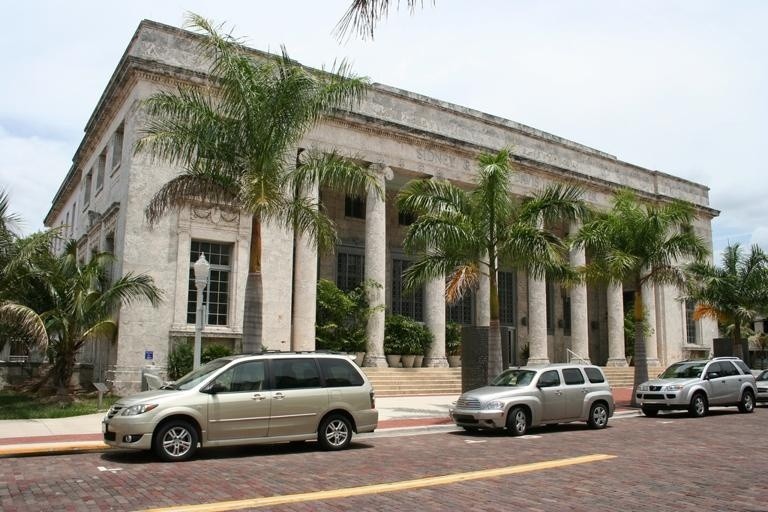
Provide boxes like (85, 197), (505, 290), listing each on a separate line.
(190, 250), (211, 373)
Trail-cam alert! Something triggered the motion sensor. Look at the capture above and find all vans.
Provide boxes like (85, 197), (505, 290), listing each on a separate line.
(448, 363), (616, 438)
(100, 348), (378, 464)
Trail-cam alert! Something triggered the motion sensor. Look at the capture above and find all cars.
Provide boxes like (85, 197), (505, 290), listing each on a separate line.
(752, 369), (767, 408)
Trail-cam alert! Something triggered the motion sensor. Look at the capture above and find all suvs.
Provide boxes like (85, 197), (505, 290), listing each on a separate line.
(634, 355), (759, 419)
